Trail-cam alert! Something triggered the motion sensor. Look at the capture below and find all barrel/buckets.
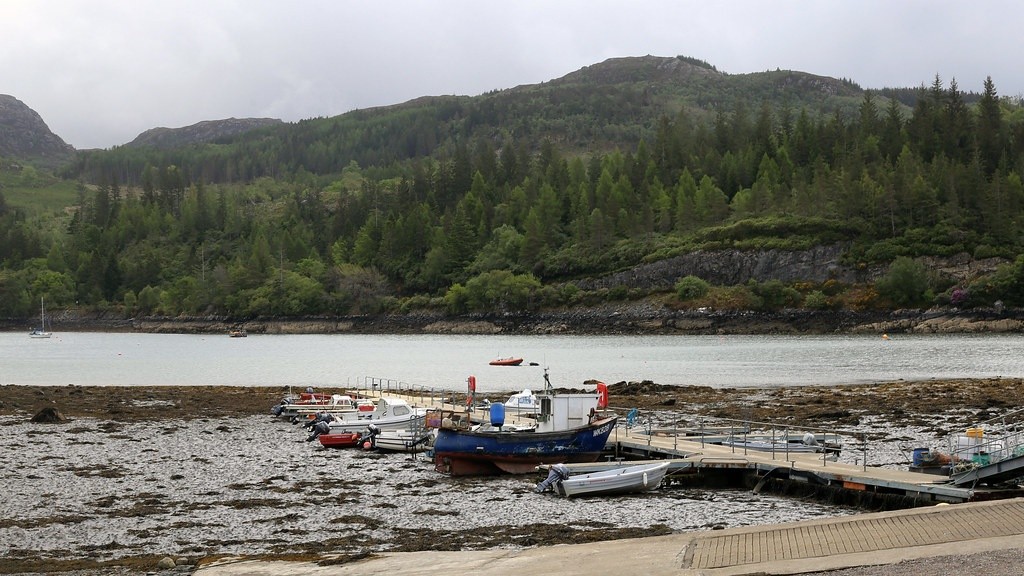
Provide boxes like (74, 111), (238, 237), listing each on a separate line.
(913, 448), (929, 466)
(973, 451), (991, 467)
(966, 428), (983, 437)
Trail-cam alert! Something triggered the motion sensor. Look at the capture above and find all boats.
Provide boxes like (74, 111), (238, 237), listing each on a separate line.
(274, 387), (536, 451)
(229, 322), (247, 337)
(726, 433), (841, 455)
(490, 353), (523, 365)
(537, 459), (670, 498)
(897, 428), (1012, 473)
(432, 355), (620, 478)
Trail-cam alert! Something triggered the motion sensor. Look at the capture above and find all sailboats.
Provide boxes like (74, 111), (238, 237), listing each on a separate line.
(28, 296), (52, 338)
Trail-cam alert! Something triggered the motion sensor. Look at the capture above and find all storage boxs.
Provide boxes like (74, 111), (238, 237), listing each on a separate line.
(967, 428), (983, 437)
